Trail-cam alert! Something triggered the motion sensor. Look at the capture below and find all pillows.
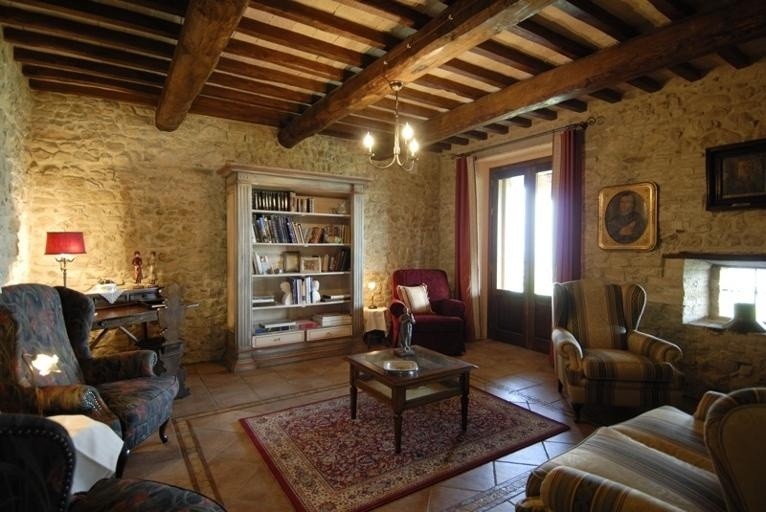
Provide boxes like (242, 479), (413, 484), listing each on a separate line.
(394, 281), (434, 317)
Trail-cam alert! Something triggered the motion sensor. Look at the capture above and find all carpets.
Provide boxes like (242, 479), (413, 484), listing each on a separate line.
(235, 378), (567, 511)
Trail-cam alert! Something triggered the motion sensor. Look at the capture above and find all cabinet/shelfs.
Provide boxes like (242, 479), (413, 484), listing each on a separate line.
(213, 159), (375, 375)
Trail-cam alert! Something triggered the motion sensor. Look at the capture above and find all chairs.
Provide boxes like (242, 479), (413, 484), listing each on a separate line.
(546, 275), (689, 427)
(0, 277), (186, 484)
(0, 412), (228, 512)
(387, 268), (468, 357)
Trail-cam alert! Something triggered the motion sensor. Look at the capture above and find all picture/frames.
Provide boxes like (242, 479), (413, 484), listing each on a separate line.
(704, 137), (764, 212)
(595, 178), (660, 254)
(297, 255), (321, 275)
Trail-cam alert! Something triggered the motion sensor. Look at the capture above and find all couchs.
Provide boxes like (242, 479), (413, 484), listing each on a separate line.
(505, 387), (765, 512)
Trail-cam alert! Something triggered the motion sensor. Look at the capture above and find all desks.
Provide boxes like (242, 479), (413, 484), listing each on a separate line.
(361, 305), (391, 350)
(42, 412), (123, 495)
(78, 285), (202, 359)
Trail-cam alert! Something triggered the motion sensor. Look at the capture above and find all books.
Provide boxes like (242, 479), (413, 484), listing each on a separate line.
(252, 188), (353, 332)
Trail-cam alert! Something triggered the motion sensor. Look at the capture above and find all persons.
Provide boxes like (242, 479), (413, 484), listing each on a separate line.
(148, 251), (158, 284)
(131, 251), (143, 283)
(607, 193), (645, 243)
(399, 306), (416, 352)
(723, 158), (756, 196)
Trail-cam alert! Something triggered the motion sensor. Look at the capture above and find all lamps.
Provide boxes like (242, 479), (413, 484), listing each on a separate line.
(15, 349), (65, 418)
(366, 281), (378, 310)
(359, 81), (422, 170)
(43, 228), (87, 293)
